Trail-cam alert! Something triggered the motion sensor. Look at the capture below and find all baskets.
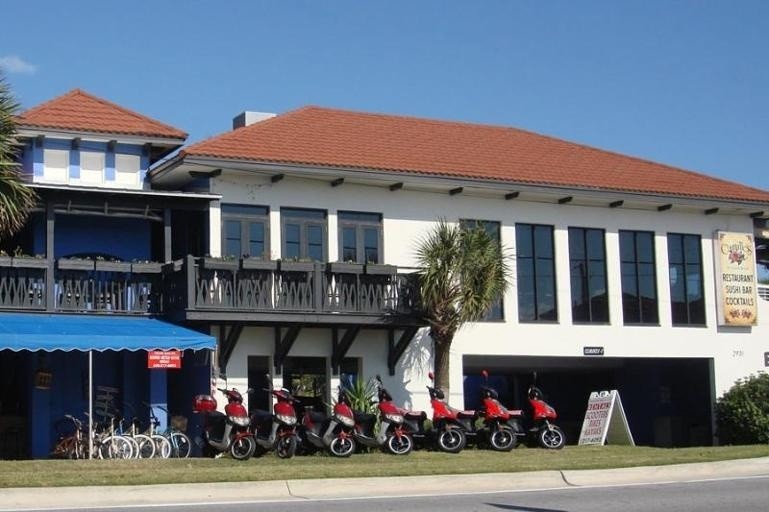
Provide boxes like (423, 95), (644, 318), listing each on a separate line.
(171, 416), (188, 431)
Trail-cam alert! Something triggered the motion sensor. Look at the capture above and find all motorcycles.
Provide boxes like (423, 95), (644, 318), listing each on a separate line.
(459, 369), (516, 449)
(192, 374), (254, 460)
(505, 371), (565, 449)
(397, 372), (465, 453)
(247, 374), (296, 458)
(350, 375), (414, 453)
(297, 373), (358, 457)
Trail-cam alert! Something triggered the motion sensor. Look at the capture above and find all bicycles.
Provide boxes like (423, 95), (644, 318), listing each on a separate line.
(54, 399), (191, 460)
